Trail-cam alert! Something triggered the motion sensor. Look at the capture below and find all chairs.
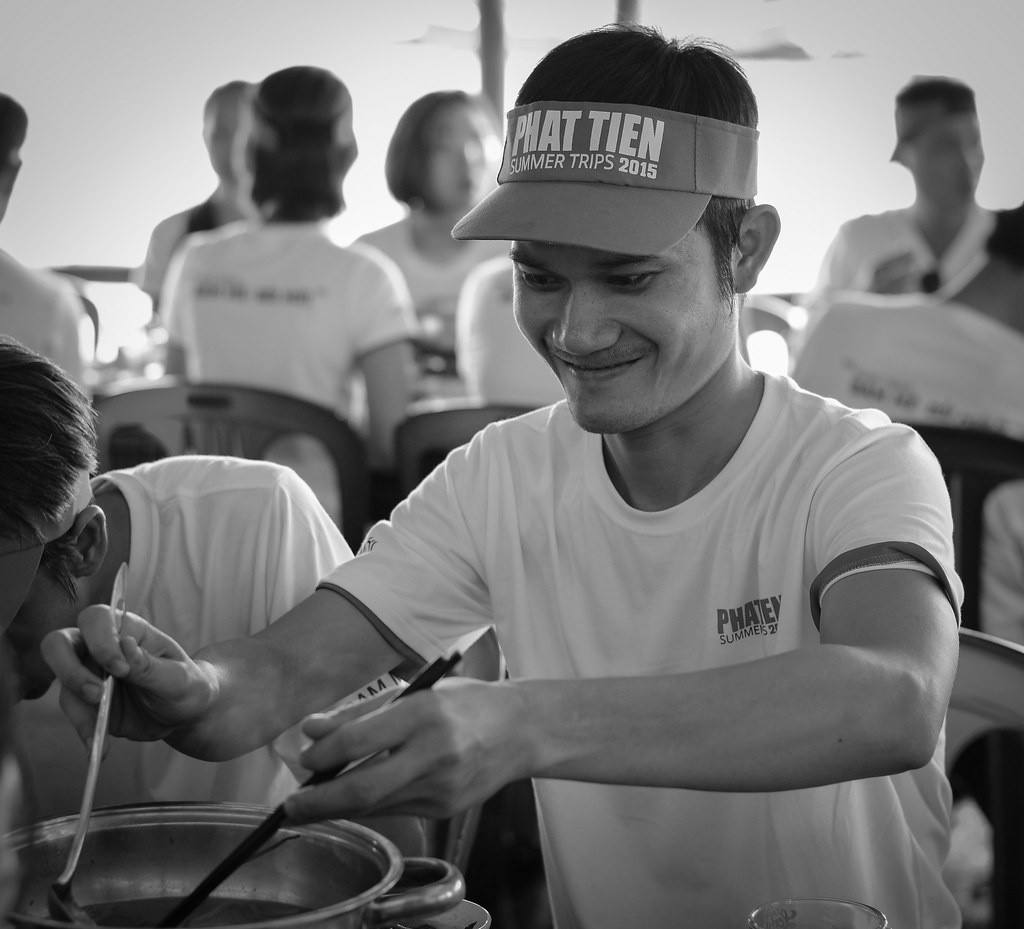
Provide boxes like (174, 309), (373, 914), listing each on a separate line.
(94, 293), (1024, 872)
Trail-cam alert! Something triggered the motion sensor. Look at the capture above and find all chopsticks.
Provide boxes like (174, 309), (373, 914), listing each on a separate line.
(158, 652), (463, 926)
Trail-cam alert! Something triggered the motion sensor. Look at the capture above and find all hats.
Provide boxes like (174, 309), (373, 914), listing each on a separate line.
(889, 76), (976, 160)
(450, 101), (759, 256)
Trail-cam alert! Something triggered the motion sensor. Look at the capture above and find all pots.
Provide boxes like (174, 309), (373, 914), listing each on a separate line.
(0, 804), (465, 929)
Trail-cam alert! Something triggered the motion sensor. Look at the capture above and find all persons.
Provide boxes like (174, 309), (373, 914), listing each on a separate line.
(43, 20), (962, 929)
(132, 66), (418, 536)
(792, 77), (1024, 659)
(1, 96), (101, 419)
(356, 91), (528, 405)
(0, 338), (434, 864)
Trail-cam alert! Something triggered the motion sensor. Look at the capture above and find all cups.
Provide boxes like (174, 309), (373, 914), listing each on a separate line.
(747, 897), (888, 929)
